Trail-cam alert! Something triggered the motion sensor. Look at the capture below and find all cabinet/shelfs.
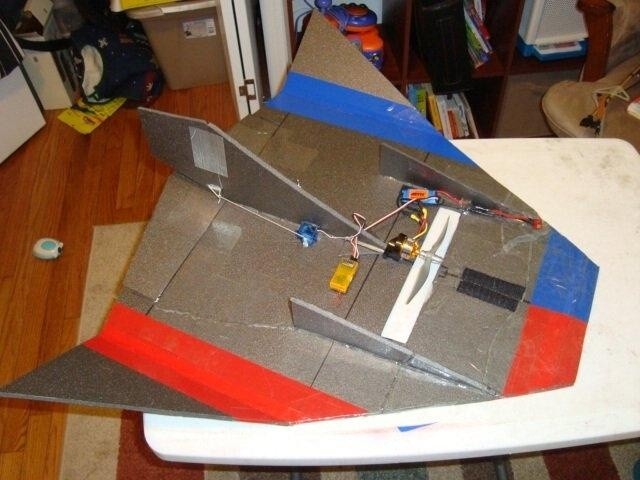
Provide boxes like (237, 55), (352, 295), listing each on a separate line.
(286, 0), (616, 139)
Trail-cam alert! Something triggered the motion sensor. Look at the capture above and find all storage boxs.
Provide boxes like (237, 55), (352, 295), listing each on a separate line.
(124, 0), (229, 91)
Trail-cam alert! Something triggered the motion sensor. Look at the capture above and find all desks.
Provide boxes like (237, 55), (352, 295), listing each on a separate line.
(142, 137), (639, 480)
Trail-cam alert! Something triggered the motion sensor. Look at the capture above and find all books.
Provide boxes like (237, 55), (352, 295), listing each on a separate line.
(410, 82), (480, 140)
(464, 0), (493, 72)
